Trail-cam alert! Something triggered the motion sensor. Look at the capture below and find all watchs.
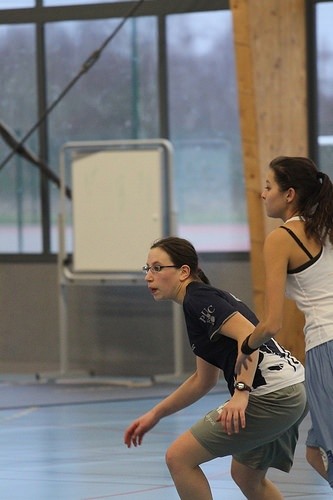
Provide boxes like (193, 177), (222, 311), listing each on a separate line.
(234, 381), (252, 392)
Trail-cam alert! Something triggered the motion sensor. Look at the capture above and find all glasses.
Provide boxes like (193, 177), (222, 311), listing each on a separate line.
(141, 264), (176, 273)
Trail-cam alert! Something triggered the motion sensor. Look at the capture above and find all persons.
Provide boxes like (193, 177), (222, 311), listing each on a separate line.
(123, 236), (310, 500)
(234, 156), (333, 487)
